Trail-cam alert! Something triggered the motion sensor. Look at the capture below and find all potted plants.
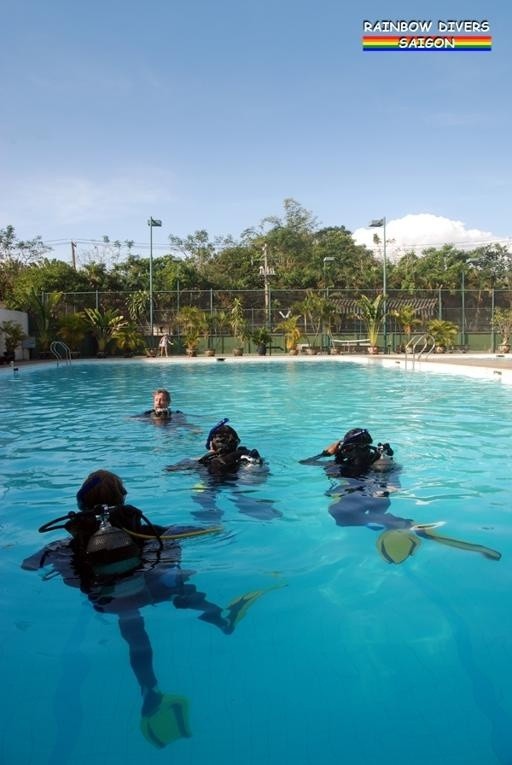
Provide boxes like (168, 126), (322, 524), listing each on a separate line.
(352, 293), (461, 355)
(0, 286), (157, 364)
(489, 306), (512, 353)
(172, 293), (342, 357)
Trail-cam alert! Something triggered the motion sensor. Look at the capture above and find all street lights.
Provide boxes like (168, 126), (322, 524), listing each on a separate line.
(148, 217), (162, 334)
(369, 218), (388, 354)
(323, 257), (335, 333)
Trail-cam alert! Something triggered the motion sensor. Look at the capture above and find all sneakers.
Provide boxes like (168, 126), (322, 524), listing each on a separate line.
(142, 690), (161, 716)
(220, 608), (238, 633)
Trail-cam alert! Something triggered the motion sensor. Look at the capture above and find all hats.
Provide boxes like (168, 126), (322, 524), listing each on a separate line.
(205, 425), (241, 457)
(344, 428), (373, 462)
(76, 470), (128, 511)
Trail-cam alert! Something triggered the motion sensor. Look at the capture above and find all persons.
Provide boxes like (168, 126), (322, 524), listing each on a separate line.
(161, 425), (283, 521)
(158, 334), (174, 357)
(129, 388), (203, 435)
(298, 427), (433, 537)
(20, 468), (232, 716)
